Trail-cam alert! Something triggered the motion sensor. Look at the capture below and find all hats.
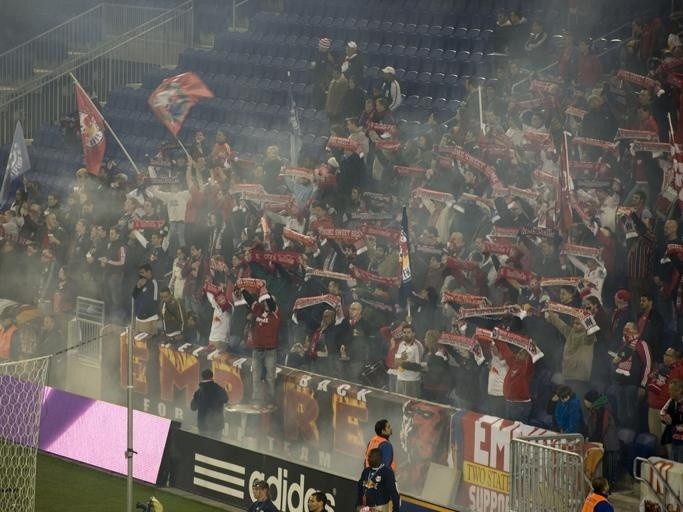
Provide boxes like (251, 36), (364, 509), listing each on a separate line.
(318, 37), (331, 49)
(380, 66), (395, 76)
(584, 390), (601, 403)
(346, 41), (357, 49)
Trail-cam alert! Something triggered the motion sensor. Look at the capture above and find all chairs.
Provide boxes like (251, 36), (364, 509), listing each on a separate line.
(0, 1), (683, 449)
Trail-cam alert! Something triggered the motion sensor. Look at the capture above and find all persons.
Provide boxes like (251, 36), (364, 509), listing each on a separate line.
(355, 449), (398, 511)
(582, 479), (614, 512)
(307, 493), (329, 512)
(248, 482), (279, 511)
